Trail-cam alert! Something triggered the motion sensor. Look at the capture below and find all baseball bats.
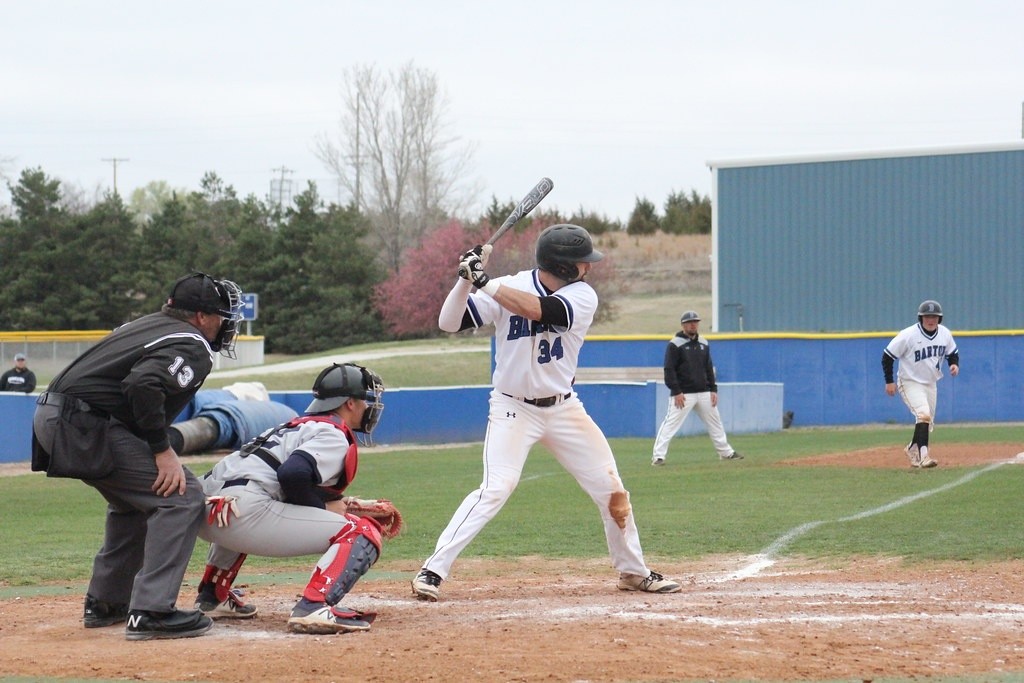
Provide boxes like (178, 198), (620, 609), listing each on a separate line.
(458, 178), (556, 276)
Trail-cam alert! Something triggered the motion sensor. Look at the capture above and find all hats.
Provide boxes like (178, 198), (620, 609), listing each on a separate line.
(14, 353), (27, 360)
(680, 310), (702, 323)
(167, 273), (240, 316)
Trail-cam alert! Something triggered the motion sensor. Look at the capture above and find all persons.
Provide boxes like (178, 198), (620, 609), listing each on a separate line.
(411, 223), (682, 600)
(0, 352), (36, 393)
(651, 311), (744, 467)
(196, 361), (401, 634)
(30, 273), (243, 641)
(881, 299), (959, 468)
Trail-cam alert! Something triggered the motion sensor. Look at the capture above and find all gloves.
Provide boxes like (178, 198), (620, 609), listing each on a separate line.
(458, 244), (492, 288)
(205, 494), (241, 528)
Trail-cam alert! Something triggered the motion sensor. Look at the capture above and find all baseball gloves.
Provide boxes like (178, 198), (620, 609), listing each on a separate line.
(344, 494), (402, 539)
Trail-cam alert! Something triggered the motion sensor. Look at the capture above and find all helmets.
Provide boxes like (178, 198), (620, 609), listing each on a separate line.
(303, 362), (386, 413)
(917, 300), (943, 316)
(535, 224), (604, 281)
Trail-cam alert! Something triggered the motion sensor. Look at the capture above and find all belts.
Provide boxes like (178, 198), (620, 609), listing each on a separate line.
(502, 393), (572, 408)
(38, 391), (92, 413)
(205, 470), (250, 489)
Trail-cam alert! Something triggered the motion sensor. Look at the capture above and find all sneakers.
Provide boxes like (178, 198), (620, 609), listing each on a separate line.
(84, 597), (131, 628)
(617, 569), (682, 593)
(287, 598), (372, 633)
(127, 609), (215, 641)
(651, 458), (665, 466)
(193, 590), (257, 620)
(412, 568), (442, 601)
(919, 456), (938, 468)
(720, 451), (744, 460)
(903, 444), (920, 467)
